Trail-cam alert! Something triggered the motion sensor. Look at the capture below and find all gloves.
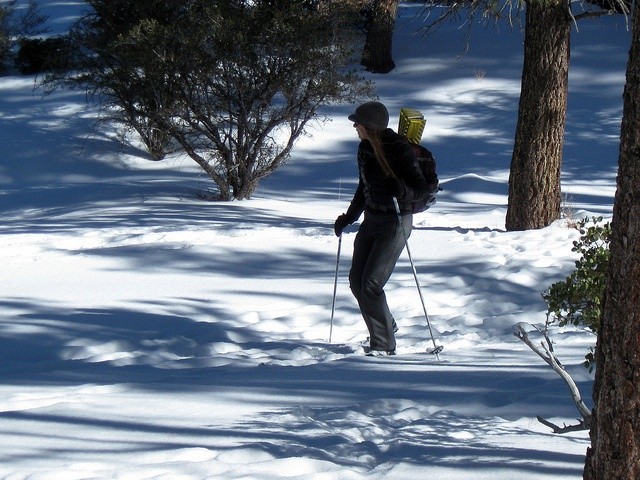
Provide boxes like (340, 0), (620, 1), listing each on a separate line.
(381, 177), (404, 202)
(334, 213), (348, 237)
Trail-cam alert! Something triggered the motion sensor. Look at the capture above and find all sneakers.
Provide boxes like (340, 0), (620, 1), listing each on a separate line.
(366, 349), (396, 357)
(364, 315), (398, 345)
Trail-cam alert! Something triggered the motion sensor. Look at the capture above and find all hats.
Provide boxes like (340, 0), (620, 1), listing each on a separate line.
(348, 102), (389, 131)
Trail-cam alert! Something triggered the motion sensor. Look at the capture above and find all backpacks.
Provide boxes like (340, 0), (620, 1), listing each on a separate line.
(411, 143), (439, 213)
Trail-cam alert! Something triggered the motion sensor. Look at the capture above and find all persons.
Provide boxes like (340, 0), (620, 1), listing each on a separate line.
(331, 100), (423, 356)
